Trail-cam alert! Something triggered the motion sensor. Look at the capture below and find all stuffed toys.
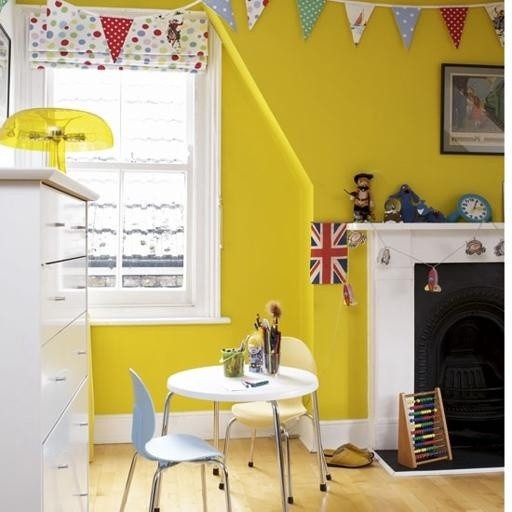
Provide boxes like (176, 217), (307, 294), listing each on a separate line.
(384, 197), (404, 223)
(246, 335), (263, 372)
(390, 183), (446, 222)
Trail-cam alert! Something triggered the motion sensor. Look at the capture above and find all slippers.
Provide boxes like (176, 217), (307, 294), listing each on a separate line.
(322, 442), (374, 467)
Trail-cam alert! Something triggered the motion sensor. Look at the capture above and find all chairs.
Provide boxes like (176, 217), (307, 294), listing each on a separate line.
(120, 337), (331, 511)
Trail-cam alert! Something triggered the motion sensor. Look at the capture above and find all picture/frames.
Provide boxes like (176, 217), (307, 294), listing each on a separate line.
(0, 23), (11, 119)
(440, 63), (503, 156)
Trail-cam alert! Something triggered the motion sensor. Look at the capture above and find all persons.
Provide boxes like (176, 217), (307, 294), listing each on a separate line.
(456, 82), (481, 130)
(349, 173), (375, 222)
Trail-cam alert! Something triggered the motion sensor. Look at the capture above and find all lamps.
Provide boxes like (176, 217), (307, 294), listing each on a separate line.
(2, 107), (114, 173)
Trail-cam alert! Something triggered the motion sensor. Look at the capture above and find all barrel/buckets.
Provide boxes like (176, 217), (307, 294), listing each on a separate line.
(220, 348), (244, 377)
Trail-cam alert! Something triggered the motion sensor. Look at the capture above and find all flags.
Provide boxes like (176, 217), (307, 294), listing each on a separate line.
(309, 221), (349, 284)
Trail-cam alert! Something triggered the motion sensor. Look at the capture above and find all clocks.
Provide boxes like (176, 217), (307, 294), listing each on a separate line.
(456, 194), (491, 223)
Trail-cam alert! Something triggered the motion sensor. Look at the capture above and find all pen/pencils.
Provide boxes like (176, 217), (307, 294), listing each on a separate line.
(255, 314), (281, 374)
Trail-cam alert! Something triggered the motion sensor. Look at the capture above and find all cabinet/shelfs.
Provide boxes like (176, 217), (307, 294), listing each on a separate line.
(0, 170), (100, 510)
